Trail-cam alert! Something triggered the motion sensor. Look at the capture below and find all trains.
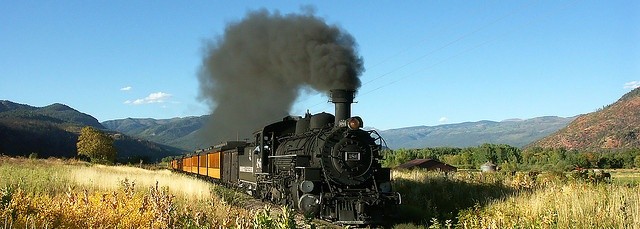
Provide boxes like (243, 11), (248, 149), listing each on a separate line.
(165, 89), (403, 226)
